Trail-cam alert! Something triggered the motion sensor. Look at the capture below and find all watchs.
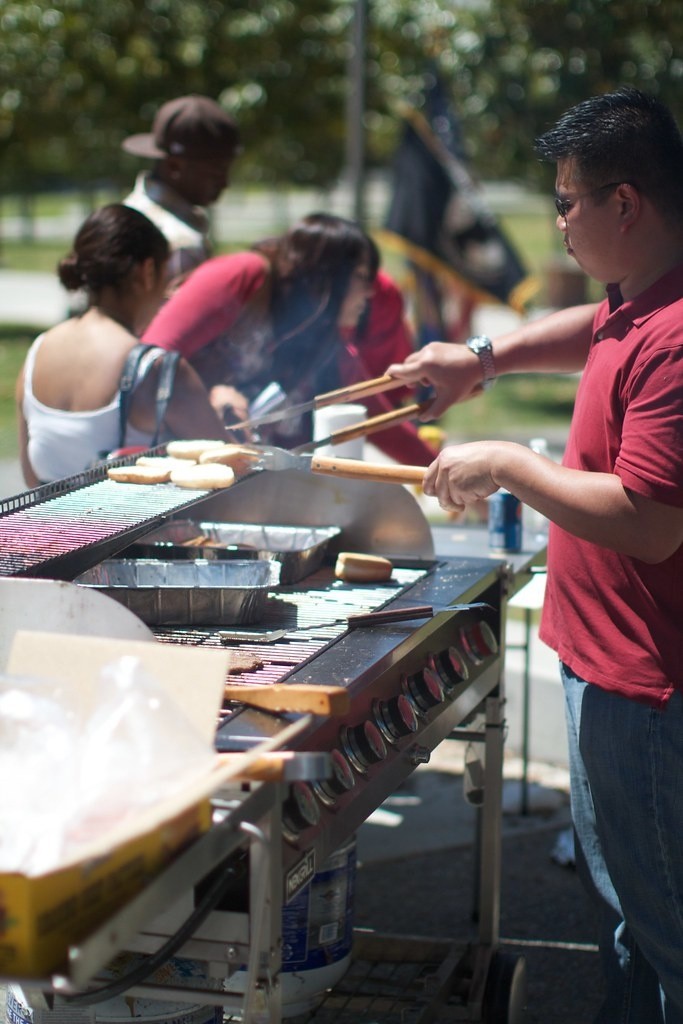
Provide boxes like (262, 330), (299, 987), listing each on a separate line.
(463, 335), (497, 388)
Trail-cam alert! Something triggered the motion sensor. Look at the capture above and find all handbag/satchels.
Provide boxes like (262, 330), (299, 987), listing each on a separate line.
(445, 191), (532, 303)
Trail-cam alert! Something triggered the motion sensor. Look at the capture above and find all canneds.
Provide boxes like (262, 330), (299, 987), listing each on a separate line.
(488, 491), (522, 552)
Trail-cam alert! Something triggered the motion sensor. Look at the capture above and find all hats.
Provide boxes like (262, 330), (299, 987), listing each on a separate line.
(120, 95), (241, 159)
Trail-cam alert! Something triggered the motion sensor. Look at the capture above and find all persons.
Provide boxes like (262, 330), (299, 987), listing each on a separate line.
(17, 86), (683, 1024)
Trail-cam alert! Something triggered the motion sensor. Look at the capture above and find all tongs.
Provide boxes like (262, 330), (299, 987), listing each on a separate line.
(224, 370), (483, 455)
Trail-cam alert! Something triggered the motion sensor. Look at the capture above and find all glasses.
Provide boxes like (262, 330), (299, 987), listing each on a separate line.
(554, 182), (620, 217)
